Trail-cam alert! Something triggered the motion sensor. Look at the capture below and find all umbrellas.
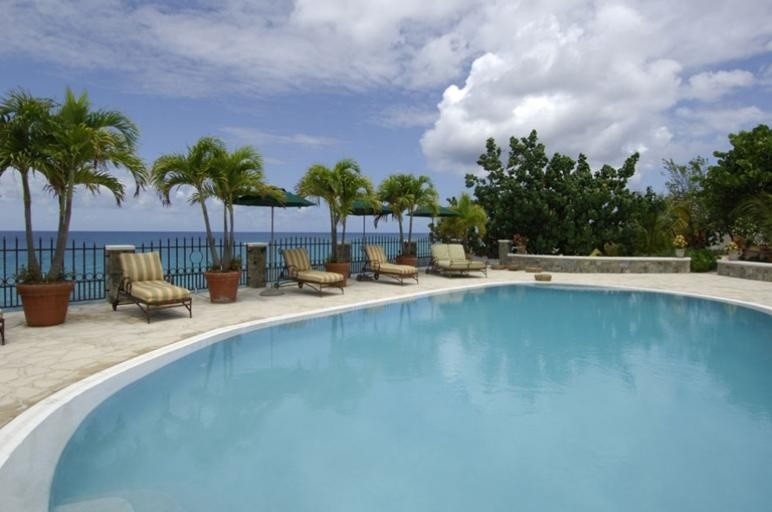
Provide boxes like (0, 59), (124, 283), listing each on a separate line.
(407, 203), (465, 244)
(232, 187), (316, 298)
(335, 199), (392, 248)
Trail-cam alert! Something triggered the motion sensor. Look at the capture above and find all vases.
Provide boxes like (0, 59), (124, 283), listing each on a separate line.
(516, 246), (526, 254)
(728, 250), (739, 260)
(675, 247), (685, 257)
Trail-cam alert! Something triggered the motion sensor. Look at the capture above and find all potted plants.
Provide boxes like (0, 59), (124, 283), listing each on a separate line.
(0, 85), (147, 327)
(293, 157), (383, 288)
(150, 136), (286, 303)
(372, 172), (441, 277)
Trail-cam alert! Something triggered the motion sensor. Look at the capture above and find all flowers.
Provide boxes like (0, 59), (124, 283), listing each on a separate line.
(672, 234), (688, 248)
(728, 242), (738, 251)
(513, 233), (529, 246)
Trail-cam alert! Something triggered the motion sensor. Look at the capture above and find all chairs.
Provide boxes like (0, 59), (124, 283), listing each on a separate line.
(426, 243), (487, 278)
(356, 244), (419, 285)
(112, 251), (193, 324)
(274, 247), (344, 296)
